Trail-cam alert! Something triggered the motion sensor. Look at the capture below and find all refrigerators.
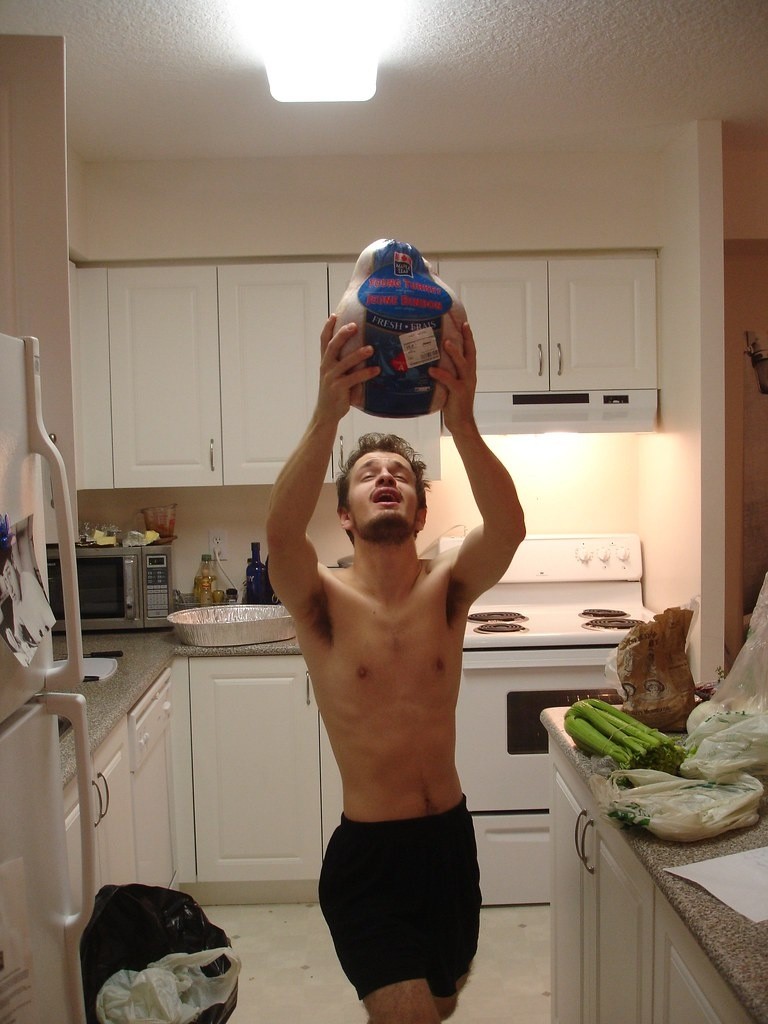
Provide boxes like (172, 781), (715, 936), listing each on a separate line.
(0, 333), (98, 1024)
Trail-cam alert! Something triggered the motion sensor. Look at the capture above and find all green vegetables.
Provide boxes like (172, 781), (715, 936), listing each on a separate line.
(564, 697), (697, 787)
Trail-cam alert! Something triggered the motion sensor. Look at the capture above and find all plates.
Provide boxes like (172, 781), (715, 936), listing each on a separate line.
(153, 536), (177, 544)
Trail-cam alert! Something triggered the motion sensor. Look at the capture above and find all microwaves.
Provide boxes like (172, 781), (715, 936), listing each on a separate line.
(46, 537), (174, 633)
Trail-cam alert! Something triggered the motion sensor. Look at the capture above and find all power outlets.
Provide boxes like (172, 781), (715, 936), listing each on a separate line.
(208, 529), (229, 561)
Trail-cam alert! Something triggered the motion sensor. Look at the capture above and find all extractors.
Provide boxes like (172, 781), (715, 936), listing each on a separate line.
(439, 391), (658, 437)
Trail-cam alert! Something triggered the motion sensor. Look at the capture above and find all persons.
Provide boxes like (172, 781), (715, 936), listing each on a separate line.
(0, 546), (57, 647)
(267, 313), (526, 1024)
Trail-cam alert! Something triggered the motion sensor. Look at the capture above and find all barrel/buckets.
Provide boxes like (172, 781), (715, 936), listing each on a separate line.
(140, 504), (175, 537)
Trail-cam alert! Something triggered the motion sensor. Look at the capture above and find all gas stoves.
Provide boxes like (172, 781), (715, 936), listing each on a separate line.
(437, 534), (661, 649)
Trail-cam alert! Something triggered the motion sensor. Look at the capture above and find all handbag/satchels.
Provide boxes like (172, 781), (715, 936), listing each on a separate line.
(600, 710), (768, 840)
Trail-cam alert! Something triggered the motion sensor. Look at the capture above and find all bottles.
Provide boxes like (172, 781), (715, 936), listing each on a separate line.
(241, 542), (279, 605)
(194, 554), (238, 610)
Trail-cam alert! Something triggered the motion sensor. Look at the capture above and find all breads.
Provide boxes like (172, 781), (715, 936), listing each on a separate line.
(92, 529), (117, 545)
(144, 530), (160, 540)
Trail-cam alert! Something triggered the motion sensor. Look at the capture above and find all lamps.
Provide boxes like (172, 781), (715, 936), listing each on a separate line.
(264, 37), (378, 102)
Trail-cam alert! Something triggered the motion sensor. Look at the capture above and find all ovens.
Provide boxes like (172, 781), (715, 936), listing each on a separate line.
(445, 645), (625, 906)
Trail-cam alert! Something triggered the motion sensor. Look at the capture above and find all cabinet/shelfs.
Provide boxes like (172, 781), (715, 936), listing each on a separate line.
(170, 655), (344, 883)
(64, 666), (176, 893)
(69, 257), (659, 489)
(548, 730), (755, 1024)
